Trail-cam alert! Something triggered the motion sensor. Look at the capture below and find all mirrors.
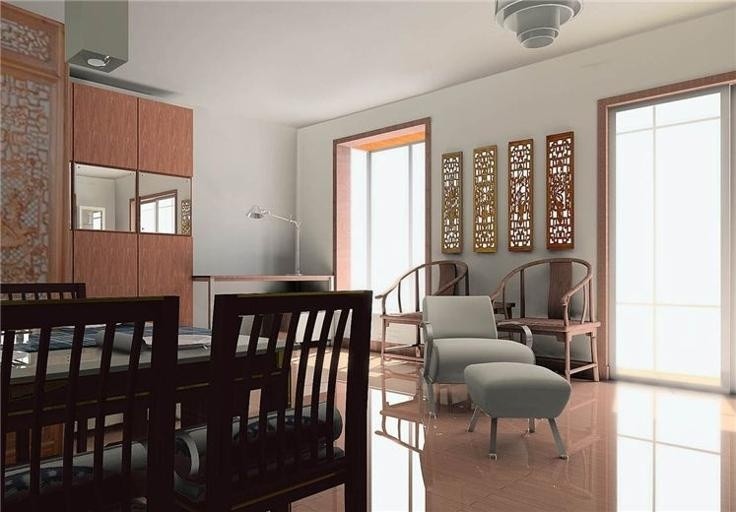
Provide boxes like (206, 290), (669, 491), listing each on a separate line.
(69, 159), (138, 237)
(137, 169), (192, 237)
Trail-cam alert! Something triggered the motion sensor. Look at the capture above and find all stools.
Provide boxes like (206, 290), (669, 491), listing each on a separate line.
(462, 361), (572, 460)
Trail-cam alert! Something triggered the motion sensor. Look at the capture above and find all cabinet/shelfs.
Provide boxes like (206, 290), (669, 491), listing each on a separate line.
(65, 80), (193, 328)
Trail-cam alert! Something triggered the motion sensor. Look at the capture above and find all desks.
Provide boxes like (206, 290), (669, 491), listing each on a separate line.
(192, 273), (334, 331)
(1, 329), (294, 512)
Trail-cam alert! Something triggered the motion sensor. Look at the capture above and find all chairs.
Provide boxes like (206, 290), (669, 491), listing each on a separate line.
(1, 295), (181, 512)
(374, 260), (470, 364)
(1, 283), (89, 463)
(174, 290), (372, 512)
(490, 257), (602, 383)
(418, 295), (535, 420)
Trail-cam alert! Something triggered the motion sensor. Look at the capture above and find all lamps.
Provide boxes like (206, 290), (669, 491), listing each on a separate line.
(249, 206), (302, 276)
(64, 0), (128, 74)
(494, 0), (582, 48)
